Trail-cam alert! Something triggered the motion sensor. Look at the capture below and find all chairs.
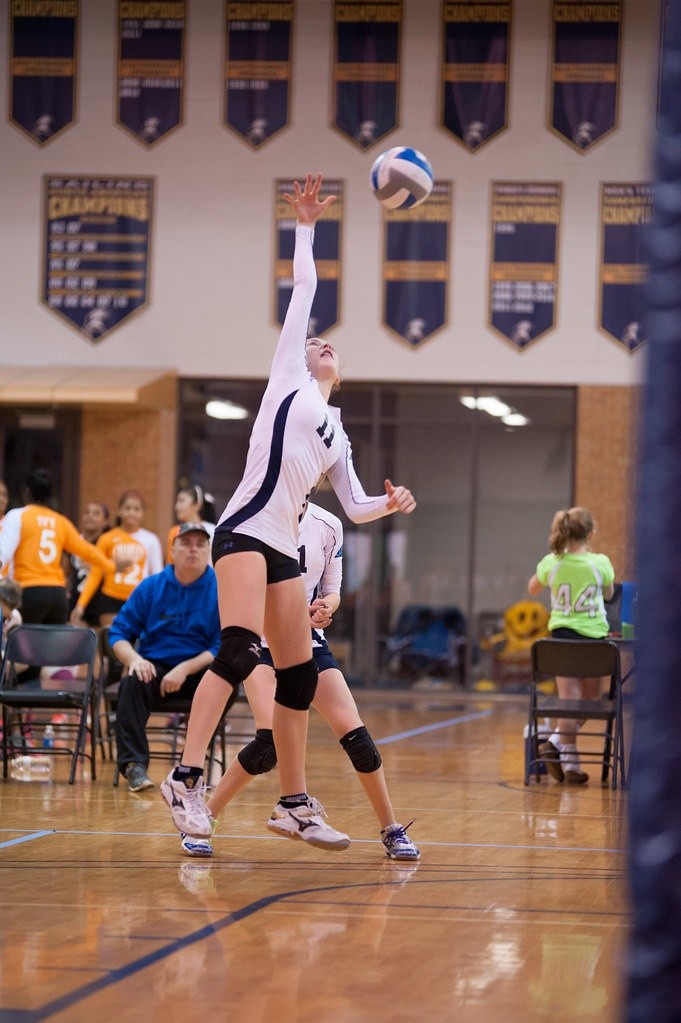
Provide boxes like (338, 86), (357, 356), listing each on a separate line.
(0, 623), (257, 790)
(524, 637), (626, 791)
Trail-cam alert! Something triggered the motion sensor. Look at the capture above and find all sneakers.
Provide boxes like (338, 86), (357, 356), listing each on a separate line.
(159, 766), (213, 838)
(267, 796), (350, 851)
(180, 816), (218, 856)
(125, 762), (154, 791)
(381, 818), (420, 860)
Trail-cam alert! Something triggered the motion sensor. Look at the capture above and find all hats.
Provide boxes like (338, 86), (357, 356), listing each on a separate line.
(172, 522), (210, 545)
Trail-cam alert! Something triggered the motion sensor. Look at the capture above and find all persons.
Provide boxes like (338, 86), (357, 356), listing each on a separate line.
(179, 473), (421, 859)
(65, 500), (112, 737)
(70, 491), (163, 732)
(165, 485), (230, 739)
(526, 505), (615, 784)
(107, 521), (239, 793)
(159, 172), (417, 852)
(0, 470), (131, 751)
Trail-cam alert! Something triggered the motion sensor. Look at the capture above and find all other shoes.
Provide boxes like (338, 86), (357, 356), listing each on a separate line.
(539, 743), (564, 781)
(565, 768), (588, 784)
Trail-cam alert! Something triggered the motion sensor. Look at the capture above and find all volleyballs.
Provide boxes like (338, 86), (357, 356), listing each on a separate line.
(370, 146), (433, 210)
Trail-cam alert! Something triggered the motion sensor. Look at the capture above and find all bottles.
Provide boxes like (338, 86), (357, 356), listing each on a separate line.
(43, 724), (54, 749)
(10, 756), (51, 772)
